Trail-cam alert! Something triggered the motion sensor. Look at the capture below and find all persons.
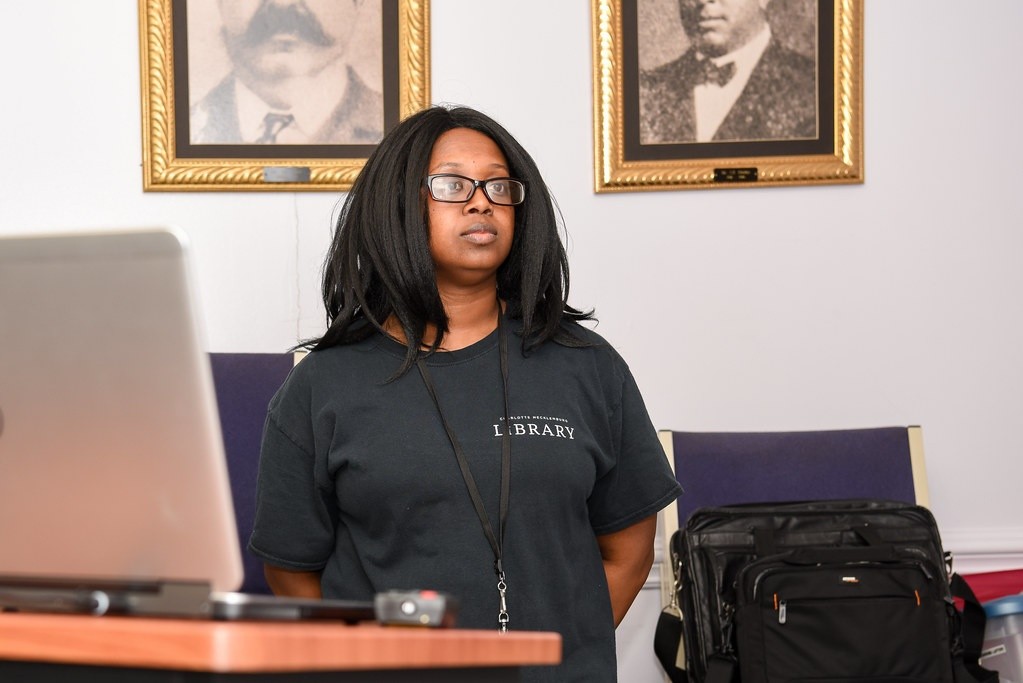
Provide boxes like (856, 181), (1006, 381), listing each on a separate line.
(639, 1), (819, 144)
(246, 109), (685, 683)
(187, 0), (385, 145)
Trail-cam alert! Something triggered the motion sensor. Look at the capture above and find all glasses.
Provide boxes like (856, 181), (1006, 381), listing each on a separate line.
(422, 174), (528, 208)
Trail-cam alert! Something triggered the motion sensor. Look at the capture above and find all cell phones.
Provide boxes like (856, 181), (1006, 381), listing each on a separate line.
(374, 592), (462, 626)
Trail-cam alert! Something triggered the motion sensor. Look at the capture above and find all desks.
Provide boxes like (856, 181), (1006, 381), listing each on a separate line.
(0, 609), (561, 683)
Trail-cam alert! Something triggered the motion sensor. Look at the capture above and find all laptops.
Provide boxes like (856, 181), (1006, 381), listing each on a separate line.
(0, 224), (375, 620)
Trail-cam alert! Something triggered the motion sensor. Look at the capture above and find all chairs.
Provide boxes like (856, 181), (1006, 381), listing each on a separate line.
(657, 425), (931, 683)
(210, 352), (309, 595)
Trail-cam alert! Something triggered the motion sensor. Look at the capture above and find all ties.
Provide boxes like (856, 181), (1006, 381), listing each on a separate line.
(256, 114), (295, 145)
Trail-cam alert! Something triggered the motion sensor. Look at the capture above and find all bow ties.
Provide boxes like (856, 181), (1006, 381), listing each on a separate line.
(691, 58), (735, 88)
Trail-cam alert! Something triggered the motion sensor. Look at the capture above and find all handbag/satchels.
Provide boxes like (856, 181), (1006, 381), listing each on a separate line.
(704, 524), (954, 683)
(653, 497), (999, 683)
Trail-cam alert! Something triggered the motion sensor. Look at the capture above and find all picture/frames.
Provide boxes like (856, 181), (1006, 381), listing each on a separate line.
(592, 0), (864, 193)
(138, 1), (430, 193)
